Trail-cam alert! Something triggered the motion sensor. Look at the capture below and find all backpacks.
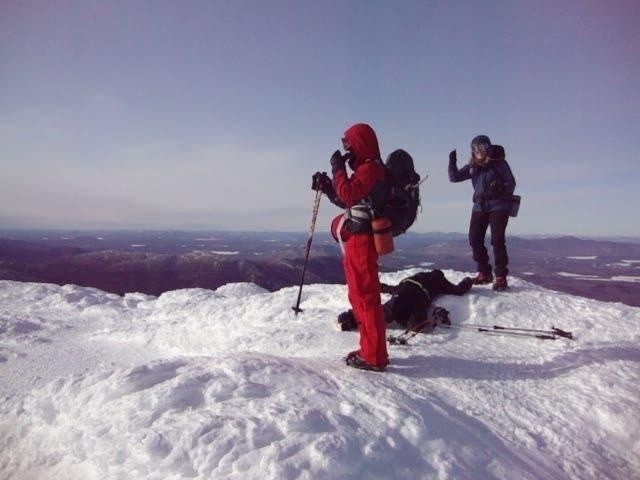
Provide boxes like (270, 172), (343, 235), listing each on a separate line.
(372, 149), (419, 236)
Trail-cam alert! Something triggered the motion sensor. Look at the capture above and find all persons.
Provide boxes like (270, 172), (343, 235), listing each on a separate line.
(335, 268), (475, 333)
(310, 122), (392, 373)
(449, 135), (515, 291)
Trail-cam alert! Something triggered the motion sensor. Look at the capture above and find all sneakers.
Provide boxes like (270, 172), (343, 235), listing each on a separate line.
(347, 350), (388, 371)
(472, 272), (506, 290)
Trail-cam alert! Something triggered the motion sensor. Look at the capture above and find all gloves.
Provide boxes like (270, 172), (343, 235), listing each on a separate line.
(330, 150), (349, 170)
(312, 172), (332, 192)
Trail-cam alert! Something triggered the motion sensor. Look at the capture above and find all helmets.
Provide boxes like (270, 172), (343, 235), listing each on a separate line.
(472, 136), (491, 152)
